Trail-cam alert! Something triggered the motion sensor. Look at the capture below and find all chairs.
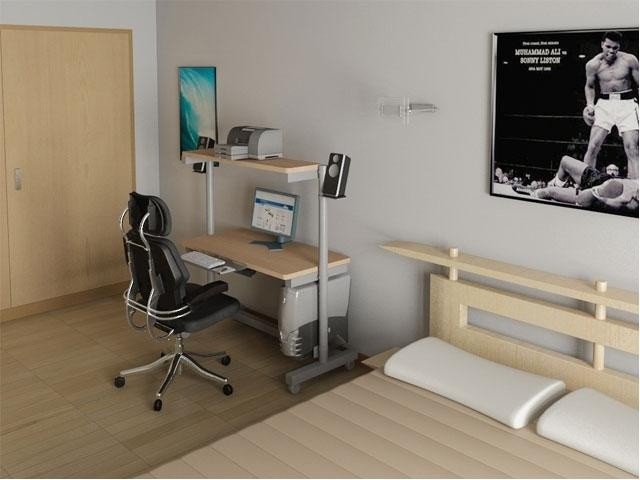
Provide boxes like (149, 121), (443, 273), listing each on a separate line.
(115, 191), (241, 411)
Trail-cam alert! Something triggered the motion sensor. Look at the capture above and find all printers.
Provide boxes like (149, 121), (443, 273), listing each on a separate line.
(214, 126), (283, 161)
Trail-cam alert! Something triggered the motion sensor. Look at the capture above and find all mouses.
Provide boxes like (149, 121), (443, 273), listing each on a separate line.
(218, 267), (231, 274)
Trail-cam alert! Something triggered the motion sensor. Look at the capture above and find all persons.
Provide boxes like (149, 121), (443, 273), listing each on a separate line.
(582, 32), (639, 180)
(512, 155), (639, 212)
(495, 161), (620, 190)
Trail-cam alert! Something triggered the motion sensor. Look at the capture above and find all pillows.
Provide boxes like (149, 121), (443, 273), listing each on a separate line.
(384, 337), (566, 430)
(536, 387), (640, 478)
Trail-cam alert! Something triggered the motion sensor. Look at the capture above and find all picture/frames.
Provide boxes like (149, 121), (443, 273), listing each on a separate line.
(490, 27), (639, 218)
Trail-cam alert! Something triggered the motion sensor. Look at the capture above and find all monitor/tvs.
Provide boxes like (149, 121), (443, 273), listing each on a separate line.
(248, 187), (299, 251)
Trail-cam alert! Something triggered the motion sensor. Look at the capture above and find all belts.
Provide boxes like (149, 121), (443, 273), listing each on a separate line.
(599, 91), (634, 101)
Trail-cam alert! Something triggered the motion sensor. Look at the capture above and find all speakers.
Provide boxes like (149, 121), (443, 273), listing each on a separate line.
(193, 136), (215, 173)
(323, 153), (351, 197)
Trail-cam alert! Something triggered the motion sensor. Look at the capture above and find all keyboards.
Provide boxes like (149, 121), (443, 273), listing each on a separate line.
(181, 251), (226, 270)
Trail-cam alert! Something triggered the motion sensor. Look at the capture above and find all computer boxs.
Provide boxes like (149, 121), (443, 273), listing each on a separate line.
(277, 272), (350, 357)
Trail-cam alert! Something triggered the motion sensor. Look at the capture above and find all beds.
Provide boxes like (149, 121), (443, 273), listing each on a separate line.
(124, 242), (639, 478)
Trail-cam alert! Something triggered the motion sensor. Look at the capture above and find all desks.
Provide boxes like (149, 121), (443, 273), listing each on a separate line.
(176, 148), (359, 393)
